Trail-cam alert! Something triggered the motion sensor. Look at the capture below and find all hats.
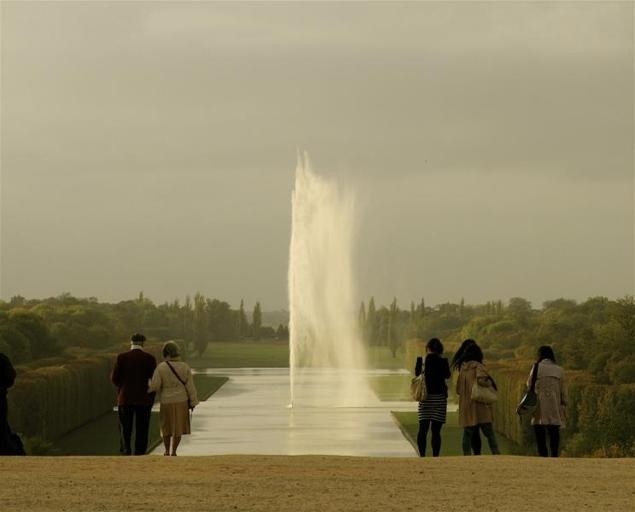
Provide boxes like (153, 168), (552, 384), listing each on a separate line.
(130, 333), (146, 341)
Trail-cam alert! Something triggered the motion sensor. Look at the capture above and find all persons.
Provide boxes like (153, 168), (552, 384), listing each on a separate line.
(414, 337), (451, 458)
(0, 352), (24, 455)
(450, 338), (501, 457)
(525, 345), (569, 458)
(456, 341), (494, 455)
(110, 330), (156, 456)
(145, 341), (199, 456)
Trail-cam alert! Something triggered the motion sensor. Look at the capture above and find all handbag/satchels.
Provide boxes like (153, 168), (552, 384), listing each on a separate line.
(470, 363), (498, 404)
(187, 397), (195, 412)
(408, 356), (428, 402)
(515, 362), (539, 416)
